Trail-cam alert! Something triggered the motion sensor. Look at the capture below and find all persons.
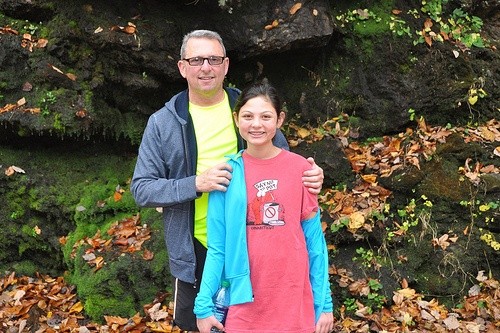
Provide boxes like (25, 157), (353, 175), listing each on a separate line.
(192, 83), (334, 333)
(130, 30), (325, 333)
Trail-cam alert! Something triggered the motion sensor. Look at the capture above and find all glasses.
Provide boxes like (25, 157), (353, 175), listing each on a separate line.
(183, 55), (225, 65)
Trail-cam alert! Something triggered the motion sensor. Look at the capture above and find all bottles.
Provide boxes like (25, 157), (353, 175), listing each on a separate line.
(210, 281), (230, 333)
